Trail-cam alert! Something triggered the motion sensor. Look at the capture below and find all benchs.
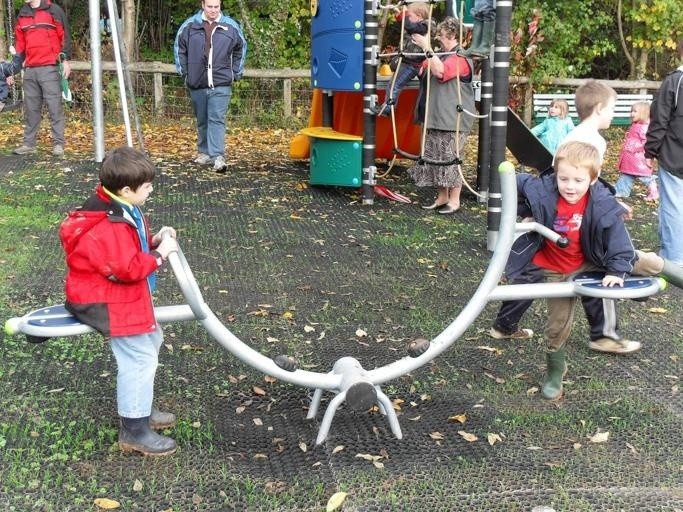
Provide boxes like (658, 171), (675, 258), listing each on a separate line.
(534, 93), (653, 137)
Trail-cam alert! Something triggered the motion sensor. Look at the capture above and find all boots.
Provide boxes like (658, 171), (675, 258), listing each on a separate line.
(118, 416), (178, 457)
(542, 347), (567, 402)
(456, 22), (482, 58)
(150, 406), (177, 429)
(472, 21), (494, 58)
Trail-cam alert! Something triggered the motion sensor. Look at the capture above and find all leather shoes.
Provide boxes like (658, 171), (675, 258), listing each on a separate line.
(438, 204), (461, 215)
(421, 200), (449, 210)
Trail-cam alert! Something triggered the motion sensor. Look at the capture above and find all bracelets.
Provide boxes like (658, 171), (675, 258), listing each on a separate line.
(422, 48), (432, 52)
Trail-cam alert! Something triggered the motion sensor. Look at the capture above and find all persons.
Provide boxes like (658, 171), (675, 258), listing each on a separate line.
(490, 80), (641, 355)
(0, 45), (25, 112)
(507, 142), (672, 404)
(12, 0), (73, 156)
(613, 102), (659, 201)
(369, 0), (433, 124)
(527, 99), (575, 156)
(642, 57), (682, 264)
(455, 0), (496, 58)
(59, 146), (179, 458)
(172, 0), (248, 172)
(418, 20), (476, 215)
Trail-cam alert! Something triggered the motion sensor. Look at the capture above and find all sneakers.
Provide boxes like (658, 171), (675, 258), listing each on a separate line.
(413, 117), (424, 125)
(489, 327), (534, 339)
(50, 144), (65, 157)
(211, 156), (227, 172)
(12, 144), (38, 156)
(643, 191), (659, 202)
(368, 105), (389, 118)
(589, 338), (641, 354)
(195, 153), (210, 165)
